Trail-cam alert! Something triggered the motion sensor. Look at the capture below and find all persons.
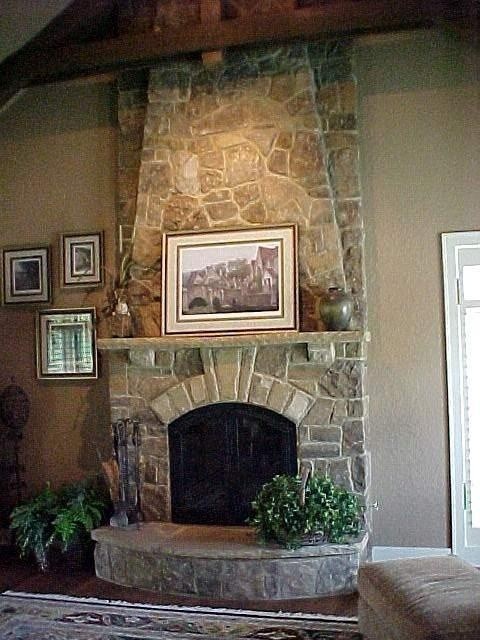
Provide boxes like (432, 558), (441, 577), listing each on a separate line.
(75, 243), (93, 275)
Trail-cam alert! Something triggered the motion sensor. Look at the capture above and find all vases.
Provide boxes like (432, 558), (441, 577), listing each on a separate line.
(319, 287), (354, 331)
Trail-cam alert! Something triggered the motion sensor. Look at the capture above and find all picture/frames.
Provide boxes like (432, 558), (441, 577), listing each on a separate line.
(35, 307), (98, 380)
(59, 230), (105, 288)
(161, 223), (300, 337)
(0, 243), (53, 308)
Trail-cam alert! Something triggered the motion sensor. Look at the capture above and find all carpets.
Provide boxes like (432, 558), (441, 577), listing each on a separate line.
(0, 590), (360, 640)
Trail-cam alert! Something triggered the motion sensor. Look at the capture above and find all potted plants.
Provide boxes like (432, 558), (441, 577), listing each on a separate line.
(77, 214), (162, 338)
(8, 469), (111, 576)
(244, 469), (367, 551)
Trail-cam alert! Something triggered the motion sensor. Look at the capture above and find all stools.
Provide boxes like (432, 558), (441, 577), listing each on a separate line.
(358, 555), (480, 640)
